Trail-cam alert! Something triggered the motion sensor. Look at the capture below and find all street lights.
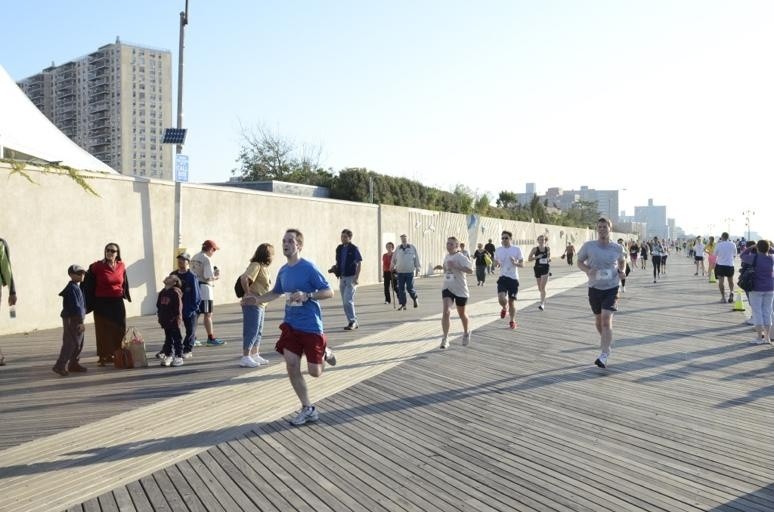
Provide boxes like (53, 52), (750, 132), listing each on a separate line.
(609, 188), (627, 232)
(742, 209), (755, 241)
(724, 216), (734, 242)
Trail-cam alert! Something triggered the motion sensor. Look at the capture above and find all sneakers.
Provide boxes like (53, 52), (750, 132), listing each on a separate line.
(501, 309), (506, 318)
(68, 364), (86, 372)
(510, 321), (516, 329)
(207, 338), (227, 346)
(414, 298), (418, 307)
(463, 331), (471, 345)
(291, 405), (318, 425)
(344, 322), (358, 330)
(746, 318), (774, 344)
(52, 365), (70, 376)
(156, 340), (201, 366)
(398, 305), (406, 310)
(326, 348), (336, 366)
(729, 292), (733, 302)
(239, 354), (268, 367)
(539, 304), (544, 311)
(595, 352), (607, 368)
(440, 339), (450, 348)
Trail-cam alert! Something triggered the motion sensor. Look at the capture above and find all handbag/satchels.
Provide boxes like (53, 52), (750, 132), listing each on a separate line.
(235, 275), (251, 297)
(483, 253), (492, 265)
(114, 347), (132, 369)
(737, 266), (755, 291)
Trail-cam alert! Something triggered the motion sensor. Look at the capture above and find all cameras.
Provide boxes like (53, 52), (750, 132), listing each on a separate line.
(328, 265), (340, 277)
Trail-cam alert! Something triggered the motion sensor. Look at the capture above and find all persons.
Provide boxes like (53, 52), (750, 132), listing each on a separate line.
(240, 244), (274, 368)
(156, 251), (201, 359)
(156, 275), (185, 366)
(78, 242), (132, 363)
(385, 215), (774, 368)
(0, 236), (18, 365)
(52, 264), (87, 376)
(239, 229), (336, 426)
(190, 238), (227, 347)
(328, 228), (363, 331)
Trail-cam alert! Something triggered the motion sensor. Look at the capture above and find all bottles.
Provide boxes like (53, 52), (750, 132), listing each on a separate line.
(8, 304), (16, 318)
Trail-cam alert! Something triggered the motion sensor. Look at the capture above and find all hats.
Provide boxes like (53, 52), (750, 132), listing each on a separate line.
(177, 252), (190, 261)
(68, 264), (88, 275)
(203, 240), (220, 250)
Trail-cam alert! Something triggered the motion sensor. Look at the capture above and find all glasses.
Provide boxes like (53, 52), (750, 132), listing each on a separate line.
(106, 249), (117, 254)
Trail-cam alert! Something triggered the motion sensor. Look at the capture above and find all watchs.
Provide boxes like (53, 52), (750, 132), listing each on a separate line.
(307, 291), (313, 300)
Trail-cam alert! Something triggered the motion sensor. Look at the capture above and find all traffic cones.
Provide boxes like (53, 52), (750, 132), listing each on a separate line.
(732, 287), (746, 311)
(709, 268), (718, 284)
